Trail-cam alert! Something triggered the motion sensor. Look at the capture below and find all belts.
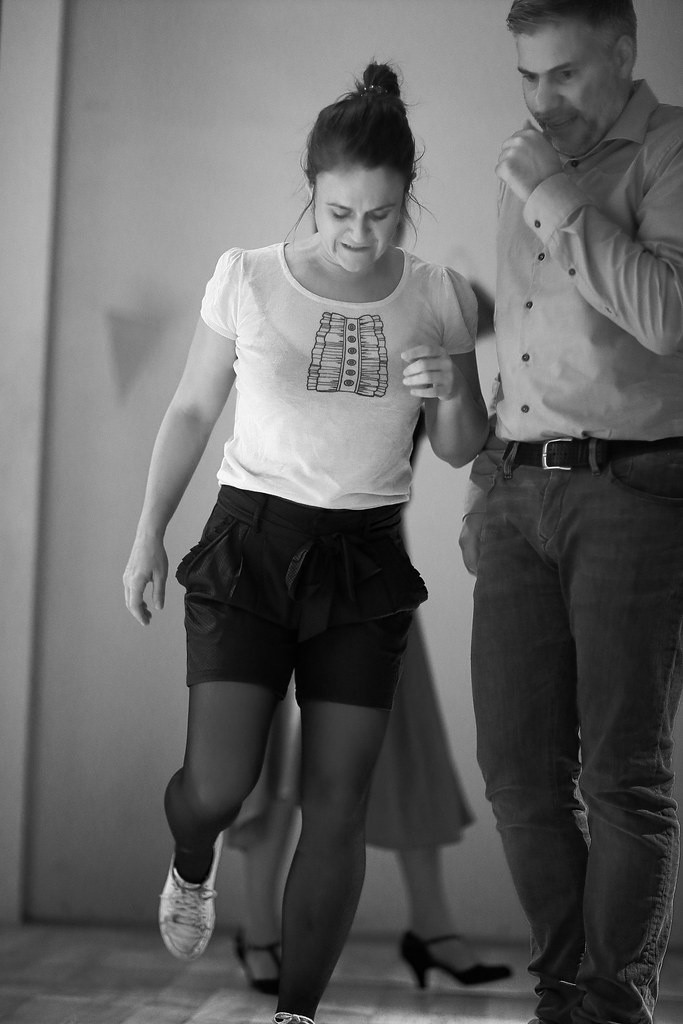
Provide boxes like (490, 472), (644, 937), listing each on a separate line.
(502, 436), (682, 471)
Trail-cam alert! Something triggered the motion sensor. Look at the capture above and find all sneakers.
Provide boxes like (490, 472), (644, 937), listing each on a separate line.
(157, 827), (225, 962)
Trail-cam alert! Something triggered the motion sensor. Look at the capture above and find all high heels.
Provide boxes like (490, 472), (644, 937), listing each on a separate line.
(232, 919), (284, 996)
(398, 925), (515, 990)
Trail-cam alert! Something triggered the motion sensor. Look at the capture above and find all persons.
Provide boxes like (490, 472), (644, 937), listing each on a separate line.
(225, 284), (513, 991)
(460, 0), (683, 1024)
(123, 63), (489, 1024)
